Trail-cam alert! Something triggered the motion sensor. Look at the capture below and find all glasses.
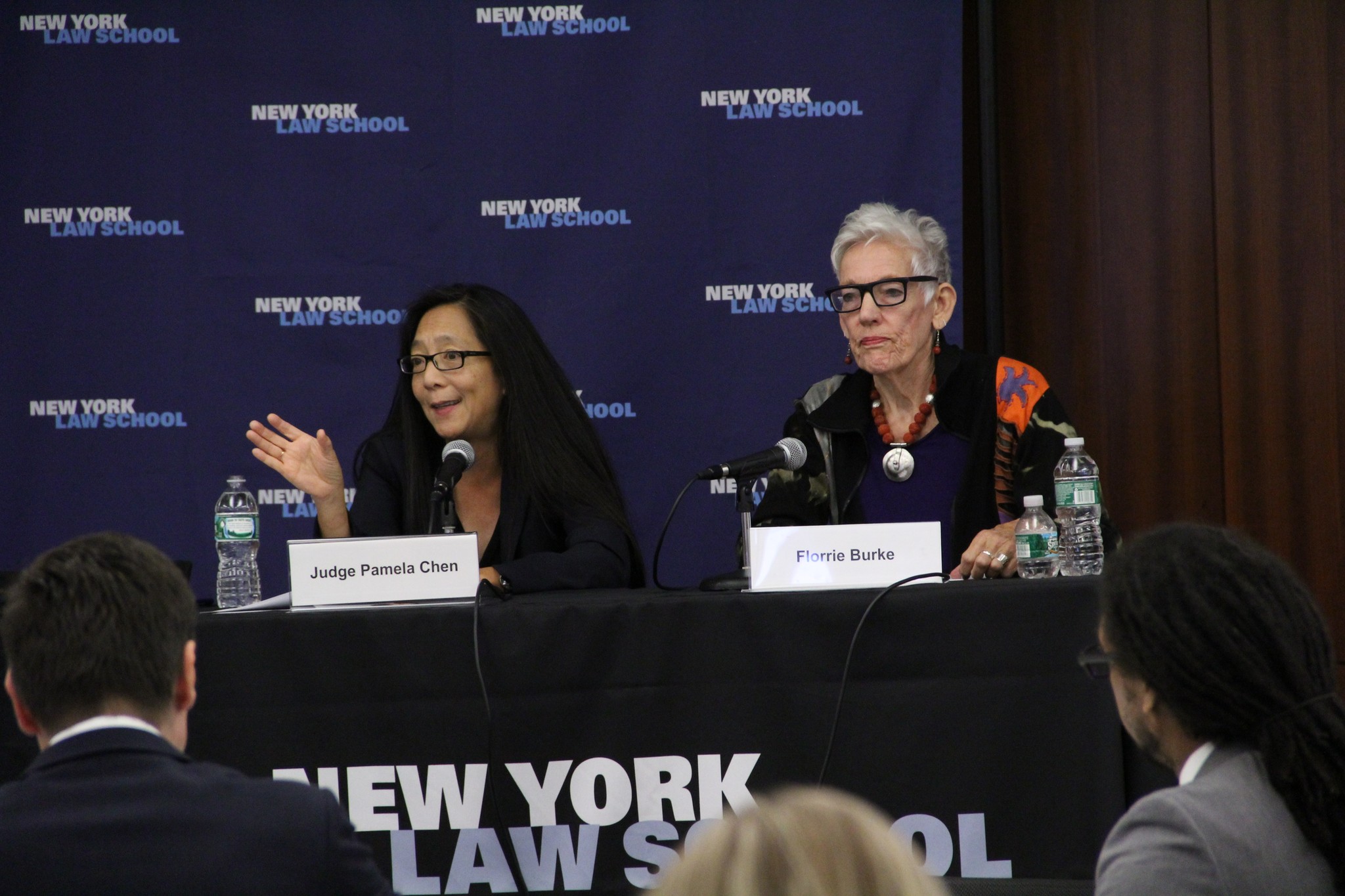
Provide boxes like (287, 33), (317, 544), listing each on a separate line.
(825, 275), (939, 313)
(1077, 652), (1127, 679)
(397, 350), (496, 374)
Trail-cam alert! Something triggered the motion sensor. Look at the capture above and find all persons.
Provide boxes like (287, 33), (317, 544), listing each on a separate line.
(1078, 522), (1345, 896)
(244, 283), (647, 596)
(648, 785), (952, 895)
(0, 533), (396, 896)
(735, 200), (1128, 583)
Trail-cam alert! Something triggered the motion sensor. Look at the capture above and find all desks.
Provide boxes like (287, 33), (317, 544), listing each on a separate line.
(187, 575), (1173, 896)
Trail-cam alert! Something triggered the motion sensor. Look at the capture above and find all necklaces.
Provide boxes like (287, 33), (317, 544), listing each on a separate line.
(870, 376), (937, 482)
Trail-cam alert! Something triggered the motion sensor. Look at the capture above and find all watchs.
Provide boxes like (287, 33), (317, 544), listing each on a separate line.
(499, 575), (511, 593)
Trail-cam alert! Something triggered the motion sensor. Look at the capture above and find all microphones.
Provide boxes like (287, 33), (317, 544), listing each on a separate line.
(696, 437), (807, 480)
(432, 439), (475, 494)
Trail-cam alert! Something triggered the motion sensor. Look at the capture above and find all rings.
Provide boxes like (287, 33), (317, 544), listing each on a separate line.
(279, 451), (284, 461)
(997, 553), (1008, 565)
(982, 550), (995, 560)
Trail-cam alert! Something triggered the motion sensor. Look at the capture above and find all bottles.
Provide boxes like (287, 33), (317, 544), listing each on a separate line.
(214, 476), (261, 608)
(1053, 437), (1104, 576)
(1014, 495), (1060, 579)
